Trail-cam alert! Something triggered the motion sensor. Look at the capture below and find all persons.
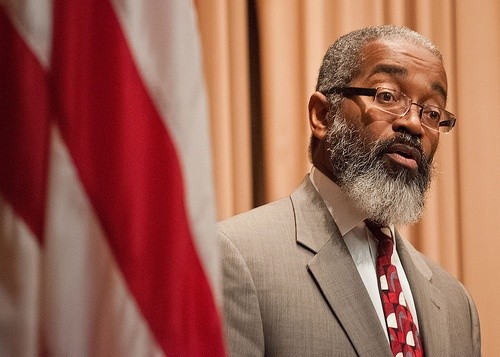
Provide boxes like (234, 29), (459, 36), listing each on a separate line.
(212, 22), (485, 355)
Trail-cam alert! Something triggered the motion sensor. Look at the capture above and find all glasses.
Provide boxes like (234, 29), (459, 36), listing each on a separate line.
(329, 87), (456, 134)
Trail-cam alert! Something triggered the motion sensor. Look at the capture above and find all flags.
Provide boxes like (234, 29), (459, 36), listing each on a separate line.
(1, 1), (228, 356)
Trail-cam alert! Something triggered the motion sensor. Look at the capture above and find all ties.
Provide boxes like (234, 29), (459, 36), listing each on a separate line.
(363, 217), (426, 357)
